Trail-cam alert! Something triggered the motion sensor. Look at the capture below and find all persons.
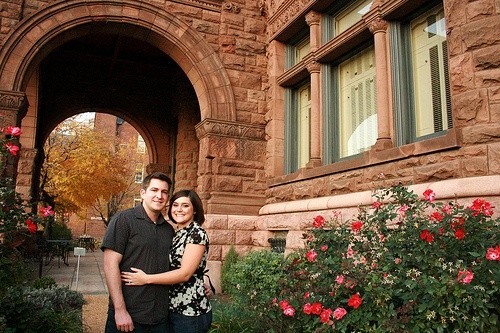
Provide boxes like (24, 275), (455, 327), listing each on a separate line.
(100, 173), (211, 333)
(122, 190), (213, 333)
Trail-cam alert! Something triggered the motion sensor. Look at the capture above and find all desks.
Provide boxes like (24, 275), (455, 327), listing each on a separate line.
(44, 240), (74, 268)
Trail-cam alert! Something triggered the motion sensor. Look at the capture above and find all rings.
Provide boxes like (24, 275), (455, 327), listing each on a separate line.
(128, 280), (130, 283)
